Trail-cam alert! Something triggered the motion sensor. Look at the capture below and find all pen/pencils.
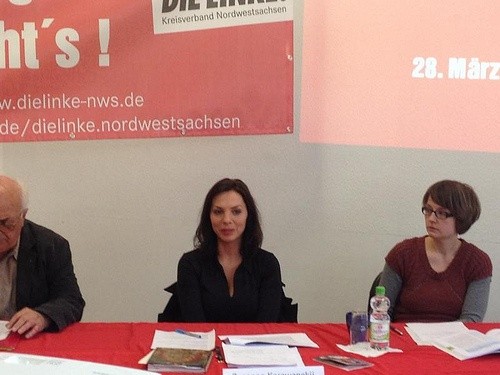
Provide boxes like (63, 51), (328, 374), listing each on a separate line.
(215, 347), (223, 363)
(390, 325), (403, 335)
(176, 329), (201, 338)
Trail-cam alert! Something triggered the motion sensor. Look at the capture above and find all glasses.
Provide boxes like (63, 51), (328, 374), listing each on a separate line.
(422, 207), (456, 219)
(0, 214), (22, 233)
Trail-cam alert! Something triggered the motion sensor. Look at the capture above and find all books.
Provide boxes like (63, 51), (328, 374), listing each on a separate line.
(147, 347), (214, 374)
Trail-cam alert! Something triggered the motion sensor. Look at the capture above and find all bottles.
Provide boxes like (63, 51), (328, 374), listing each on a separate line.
(369, 286), (390, 351)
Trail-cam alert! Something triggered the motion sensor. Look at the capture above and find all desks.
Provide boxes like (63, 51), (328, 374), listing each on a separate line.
(0, 322), (500, 375)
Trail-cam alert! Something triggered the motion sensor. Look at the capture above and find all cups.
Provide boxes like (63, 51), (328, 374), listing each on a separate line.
(346, 309), (368, 344)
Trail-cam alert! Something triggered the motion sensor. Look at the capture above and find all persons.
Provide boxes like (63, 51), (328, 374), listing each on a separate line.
(378, 179), (493, 323)
(0, 177), (86, 339)
(177, 178), (283, 324)
